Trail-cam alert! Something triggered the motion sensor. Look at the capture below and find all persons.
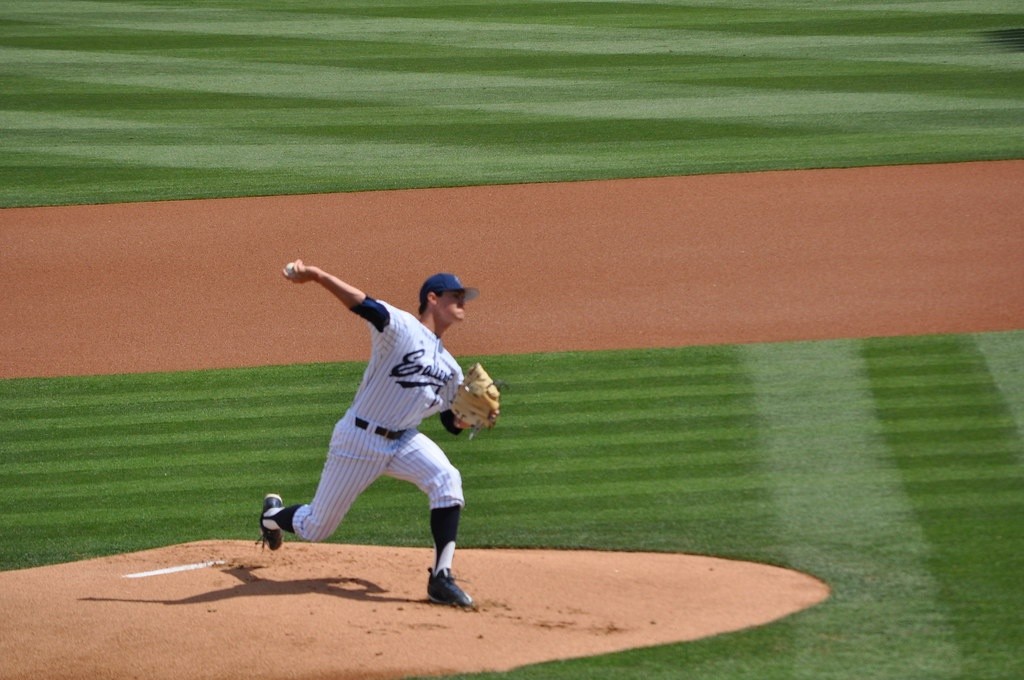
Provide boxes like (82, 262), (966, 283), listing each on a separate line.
(260, 259), (499, 606)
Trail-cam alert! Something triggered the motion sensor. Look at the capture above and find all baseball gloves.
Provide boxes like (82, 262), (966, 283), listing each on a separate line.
(451, 361), (501, 433)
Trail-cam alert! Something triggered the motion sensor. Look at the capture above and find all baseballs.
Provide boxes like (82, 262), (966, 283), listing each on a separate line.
(286, 262), (295, 274)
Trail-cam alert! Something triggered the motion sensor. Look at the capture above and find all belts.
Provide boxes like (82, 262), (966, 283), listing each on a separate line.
(355, 417), (407, 440)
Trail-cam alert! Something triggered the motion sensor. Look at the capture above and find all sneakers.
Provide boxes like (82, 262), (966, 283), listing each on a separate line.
(256, 494), (284, 551)
(427, 568), (473, 607)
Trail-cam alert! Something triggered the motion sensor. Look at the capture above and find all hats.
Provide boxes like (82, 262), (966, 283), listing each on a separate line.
(419, 273), (479, 302)
